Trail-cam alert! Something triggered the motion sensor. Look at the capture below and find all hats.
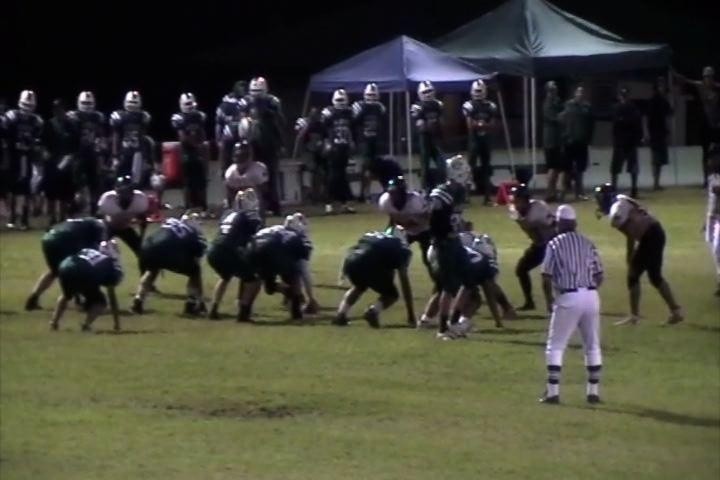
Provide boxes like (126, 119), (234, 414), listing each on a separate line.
(617, 85), (630, 95)
(655, 76), (666, 87)
(544, 80), (557, 91)
(556, 204), (576, 220)
(574, 87), (583, 96)
(703, 66), (713, 77)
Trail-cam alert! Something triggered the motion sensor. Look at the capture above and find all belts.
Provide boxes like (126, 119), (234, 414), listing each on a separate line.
(560, 286), (596, 293)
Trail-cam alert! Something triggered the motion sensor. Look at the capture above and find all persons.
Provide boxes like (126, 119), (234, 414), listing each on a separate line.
(24, 177), (324, 340)
(410, 79), (499, 207)
(670, 68), (720, 186)
(332, 155), (512, 342)
(701, 144), (719, 297)
(506, 185), (557, 312)
(608, 85), (644, 198)
(558, 85), (597, 200)
(293, 83), (389, 216)
(634, 84), (676, 191)
(538, 203), (604, 405)
(537, 81), (563, 202)
(596, 182), (685, 328)
(1, 74), (288, 230)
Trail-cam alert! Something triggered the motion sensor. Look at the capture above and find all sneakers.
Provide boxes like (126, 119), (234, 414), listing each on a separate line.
(664, 309), (686, 325)
(24, 294), (144, 331)
(546, 192), (588, 202)
(325, 202), (356, 215)
(332, 308), (380, 328)
(537, 392), (560, 403)
(614, 316), (641, 327)
(5, 214), (28, 231)
(416, 303), (535, 341)
(586, 390), (599, 403)
(183, 295), (321, 323)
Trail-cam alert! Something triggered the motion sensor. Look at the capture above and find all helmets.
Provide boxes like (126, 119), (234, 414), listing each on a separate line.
(385, 224), (408, 244)
(72, 194), (87, 209)
(417, 81), (436, 101)
(386, 177), (405, 193)
(231, 140), (250, 164)
(149, 172), (166, 192)
(473, 234), (498, 259)
(233, 77), (269, 99)
(17, 89), (37, 112)
(470, 79), (487, 101)
(114, 176), (133, 192)
(123, 91), (142, 112)
(98, 238), (120, 259)
(237, 115), (257, 141)
(181, 211), (201, 230)
(509, 183), (528, 201)
(593, 182), (615, 208)
(363, 83), (379, 100)
(49, 97), (62, 111)
(95, 214), (111, 233)
(331, 89), (349, 106)
(446, 155), (473, 185)
(77, 91), (95, 114)
(284, 213), (308, 233)
(235, 188), (259, 211)
(319, 139), (338, 159)
(178, 93), (198, 114)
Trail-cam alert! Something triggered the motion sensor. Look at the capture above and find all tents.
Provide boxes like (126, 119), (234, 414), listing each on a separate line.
(292, 36), (516, 191)
